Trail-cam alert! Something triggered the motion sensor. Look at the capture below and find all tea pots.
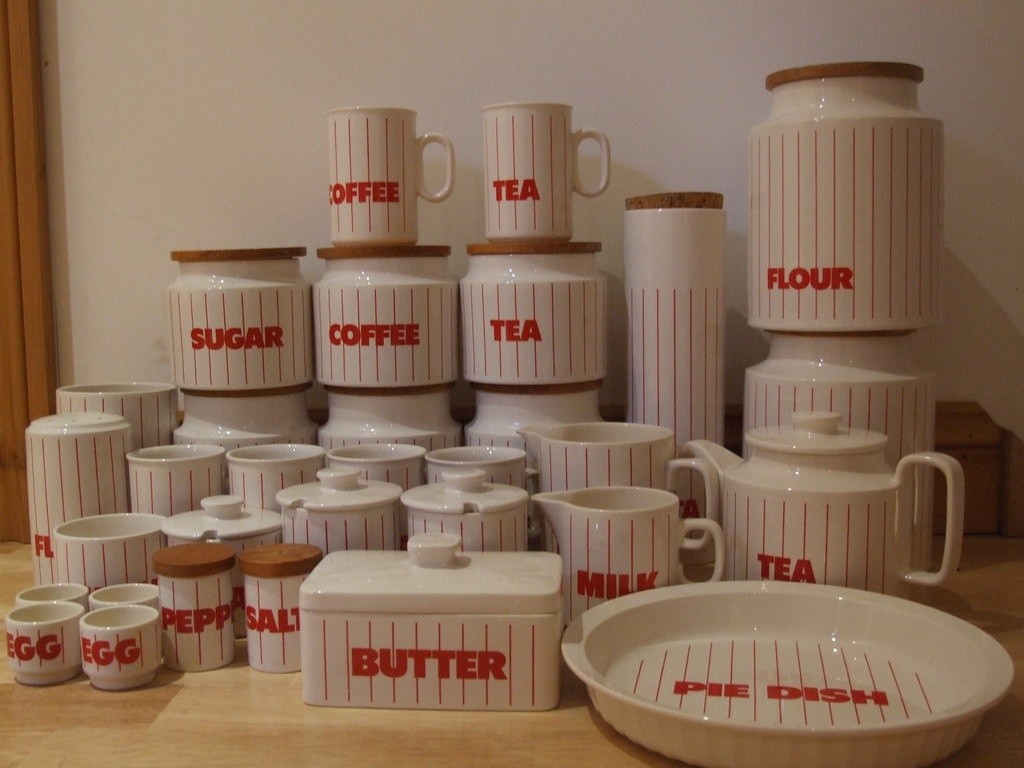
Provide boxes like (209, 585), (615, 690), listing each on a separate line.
(680, 410), (965, 606)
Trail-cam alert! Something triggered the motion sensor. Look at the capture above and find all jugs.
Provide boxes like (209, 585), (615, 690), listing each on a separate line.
(526, 489), (727, 629)
(514, 422), (719, 548)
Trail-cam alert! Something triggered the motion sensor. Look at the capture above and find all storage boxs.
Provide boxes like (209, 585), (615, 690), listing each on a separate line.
(298, 549), (563, 713)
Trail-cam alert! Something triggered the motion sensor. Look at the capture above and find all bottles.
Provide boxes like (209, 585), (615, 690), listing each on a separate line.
(274, 466), (403, 575)
(21, 415), (130, 591)
(620, 187), (728, 498)
(739, 325), (939, 595)
(148, 539), (239, 672)
(741, 63), (942, 335)
(163, 492), (284, 640)
(168, 245), (609, 546)
(234, 541), (327, 674)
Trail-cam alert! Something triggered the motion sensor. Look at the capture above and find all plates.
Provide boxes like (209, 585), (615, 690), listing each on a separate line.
(557, 578), (1013, 767)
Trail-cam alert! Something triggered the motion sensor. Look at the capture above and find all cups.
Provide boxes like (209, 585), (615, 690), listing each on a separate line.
(53, 379), (179, 454)
(13, 580), (88, 615)
(87, 581), (166, 655)
(121, 439), (425, 504)
(397, 468), (533, 554)
(477, 98), (610, 243)
(421, 445), (527, 485)
(79, 604), (165, 692)
(320, 104), (455, 247)
(52, 511), (169, 596)
(3, 603), (89, 685)
